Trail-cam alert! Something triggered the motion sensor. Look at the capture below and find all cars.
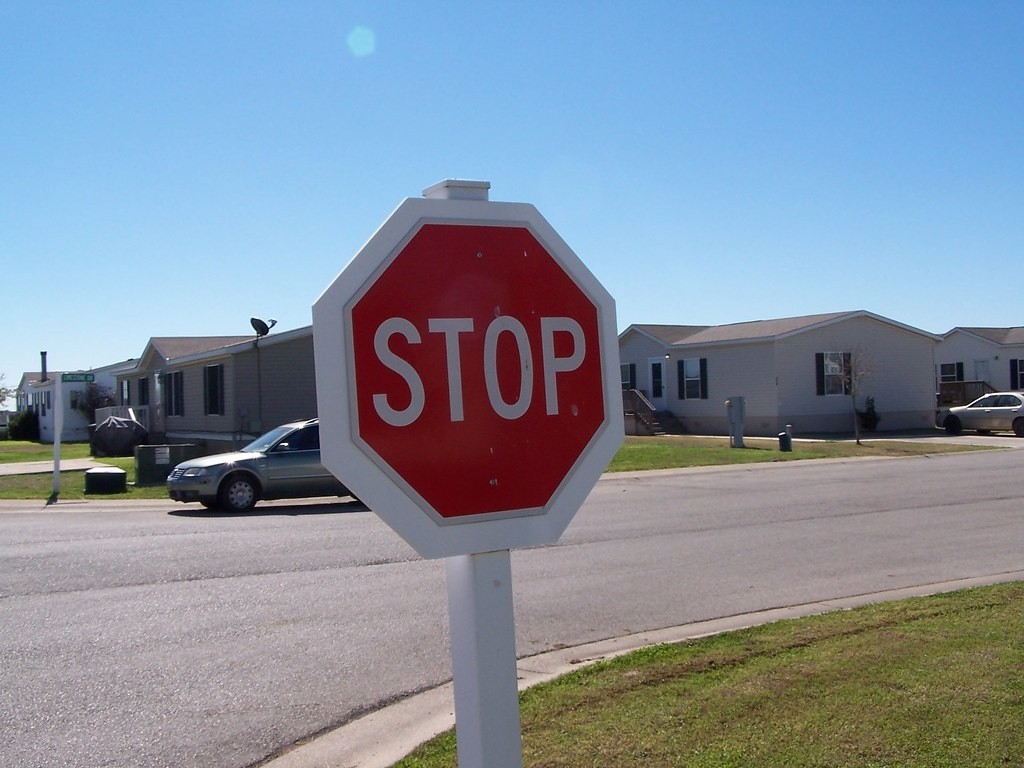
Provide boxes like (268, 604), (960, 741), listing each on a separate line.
(166, 417), (356, 514)
(935, 391), (1024, 437)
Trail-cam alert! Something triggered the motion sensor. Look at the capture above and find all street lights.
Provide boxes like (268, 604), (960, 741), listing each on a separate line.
(250, 317), (277, 436)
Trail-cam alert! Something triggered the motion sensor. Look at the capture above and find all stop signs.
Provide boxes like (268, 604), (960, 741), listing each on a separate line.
(310, 196), (625, 558)
